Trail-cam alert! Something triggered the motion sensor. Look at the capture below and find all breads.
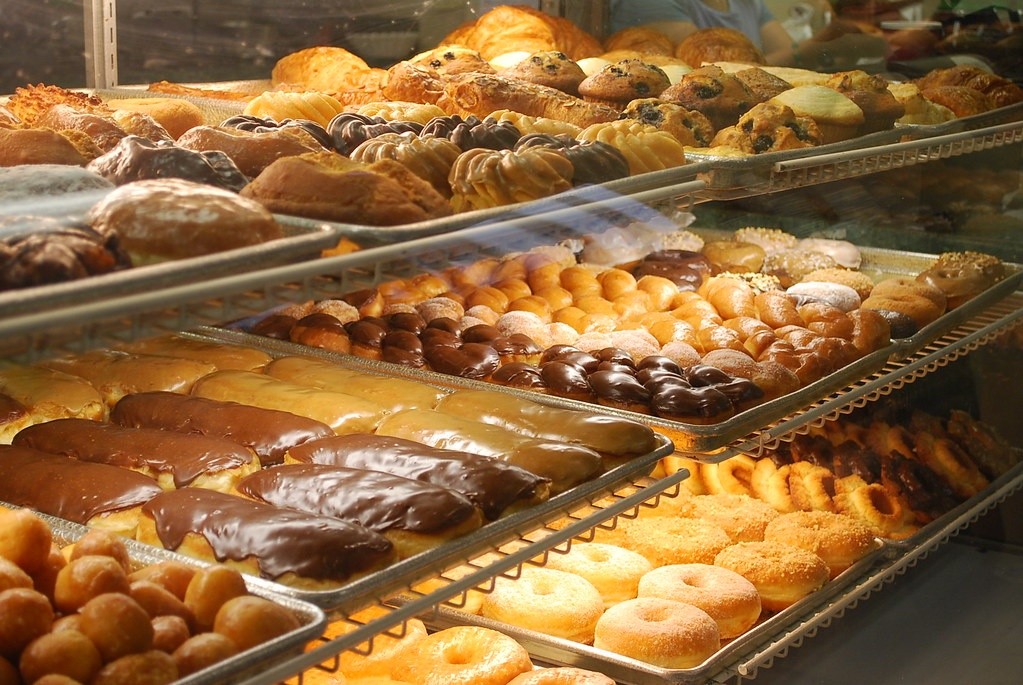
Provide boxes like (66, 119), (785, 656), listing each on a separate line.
(2, 250), (914, 580)
(0, 0), (1023, 290)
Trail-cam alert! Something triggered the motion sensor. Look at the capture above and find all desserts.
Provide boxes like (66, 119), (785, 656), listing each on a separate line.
(0, 507), (303, 685)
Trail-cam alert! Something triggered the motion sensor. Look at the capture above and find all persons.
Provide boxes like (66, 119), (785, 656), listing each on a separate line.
(609, 0), (798, 66)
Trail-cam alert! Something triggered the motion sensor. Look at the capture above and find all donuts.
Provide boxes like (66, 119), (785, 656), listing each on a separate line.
(274, 394), (1014, 685)
(552, 162), (1022, 341)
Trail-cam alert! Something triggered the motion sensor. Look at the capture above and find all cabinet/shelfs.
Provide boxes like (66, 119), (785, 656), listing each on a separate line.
(0, 120), (1023, 685)
